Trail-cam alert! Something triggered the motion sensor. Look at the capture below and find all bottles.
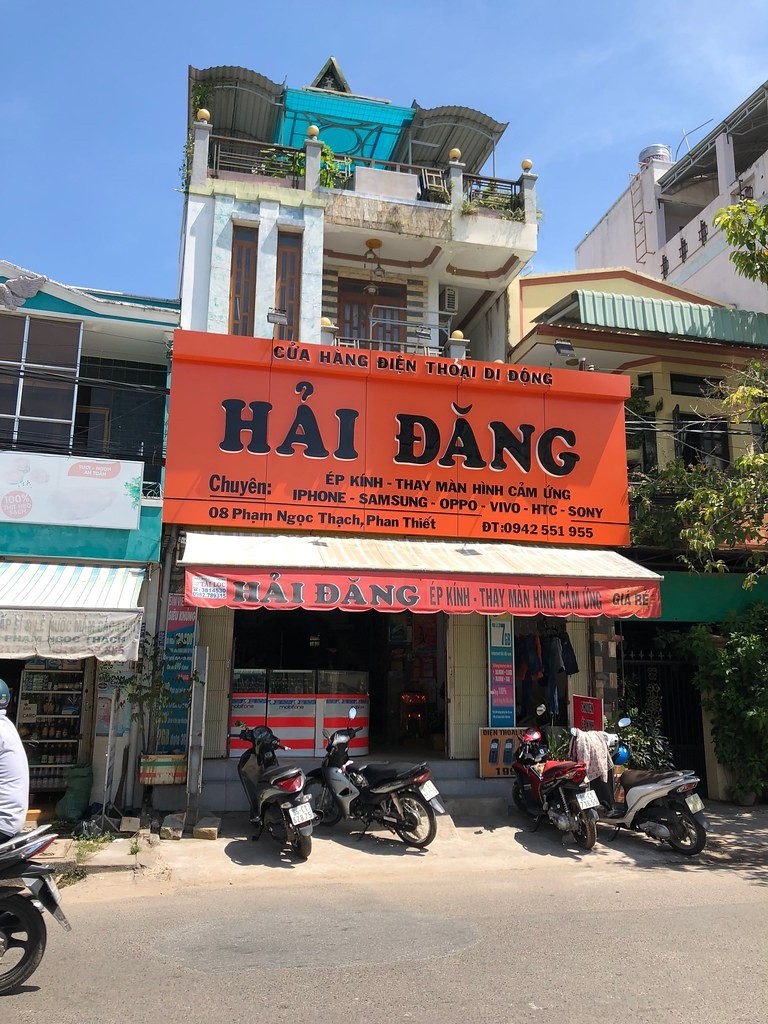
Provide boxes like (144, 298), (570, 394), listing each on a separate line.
(30, 767), (69, 788)
(62, 744), (67, 764)
(41, 744), (48, 764)
(50, 695), (55, 715)
(44, 695), (49, 703)
(56, 719), (62, 738)
(48, 744), (55, 764)
(42, 718), (48, 739)
(0, 694), (8, 709)
(67, 744), (72, 763)
(56, 694), (62, 714)
(62, 718), (69, 738)
(43, 699), (49, 714)
(55, 743), (62, 763)
(49, 719), (55, 738)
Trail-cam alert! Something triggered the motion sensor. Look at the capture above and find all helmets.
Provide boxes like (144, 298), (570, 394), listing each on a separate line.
(523, 727), (541, 741)
(0, 678), (11, 707)
(612, 746), (628, 765)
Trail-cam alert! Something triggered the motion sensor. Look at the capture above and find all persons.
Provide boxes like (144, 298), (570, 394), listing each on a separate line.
(0, 678), (30, 844)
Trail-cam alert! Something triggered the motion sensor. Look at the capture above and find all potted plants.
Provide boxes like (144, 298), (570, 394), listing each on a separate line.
(97, 630), (207, 785)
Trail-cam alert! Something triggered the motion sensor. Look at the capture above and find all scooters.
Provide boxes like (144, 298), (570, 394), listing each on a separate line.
(227, 720), (322, 857)
(570, 716), (715, 856)
(511, 704), (600, 850)
(0, 822), (73, 997)
(303, 707), (445, 848)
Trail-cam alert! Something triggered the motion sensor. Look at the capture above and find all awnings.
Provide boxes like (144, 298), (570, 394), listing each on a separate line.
(177, 531), (664, 620)
(0, 556), (146, 662)
